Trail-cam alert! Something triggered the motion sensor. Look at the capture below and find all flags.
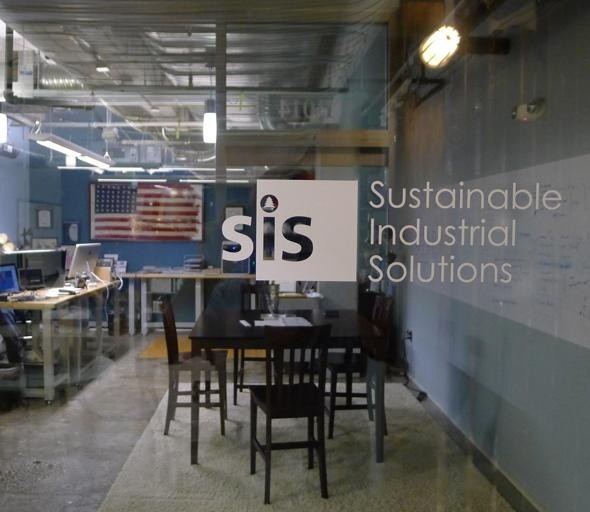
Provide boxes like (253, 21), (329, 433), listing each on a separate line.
(90, 182), (203, 242)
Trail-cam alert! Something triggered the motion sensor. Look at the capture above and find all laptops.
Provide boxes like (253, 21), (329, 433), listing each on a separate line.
(19, 269), (47, 289)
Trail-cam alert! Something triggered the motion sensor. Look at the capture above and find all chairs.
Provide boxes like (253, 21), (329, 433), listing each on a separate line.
(248, 324), (333, 504)
(234, 283), (280, 406)
(159, 294), (228, 436)
(323, 295), (391, 438)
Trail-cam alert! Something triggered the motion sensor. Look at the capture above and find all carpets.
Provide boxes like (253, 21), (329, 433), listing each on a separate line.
(98, 381), (508, 511)
(139, 336), (274, 360)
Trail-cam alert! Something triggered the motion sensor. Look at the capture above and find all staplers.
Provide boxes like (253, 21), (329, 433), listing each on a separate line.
(58, 289), (77, 295)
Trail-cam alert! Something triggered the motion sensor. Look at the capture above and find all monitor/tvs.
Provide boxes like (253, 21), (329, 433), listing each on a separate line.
(0, 263), (21, 293)
(68, 243), (102, 280)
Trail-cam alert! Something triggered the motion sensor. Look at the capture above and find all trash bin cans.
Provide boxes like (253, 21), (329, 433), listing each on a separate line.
(107, 312), (123, 336)
(0, 363), (20, 414)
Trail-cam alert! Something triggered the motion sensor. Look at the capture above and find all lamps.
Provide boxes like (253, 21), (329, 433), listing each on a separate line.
(29, 134), (110, 172)
(408, 24), (460, 84)
(203, 46), (218, 144)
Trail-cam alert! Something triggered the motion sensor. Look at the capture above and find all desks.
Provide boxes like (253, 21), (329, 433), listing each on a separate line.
(0, 273), (122, 406)
(118, 268), (256, 336)
(188, 278), (392, 464)
